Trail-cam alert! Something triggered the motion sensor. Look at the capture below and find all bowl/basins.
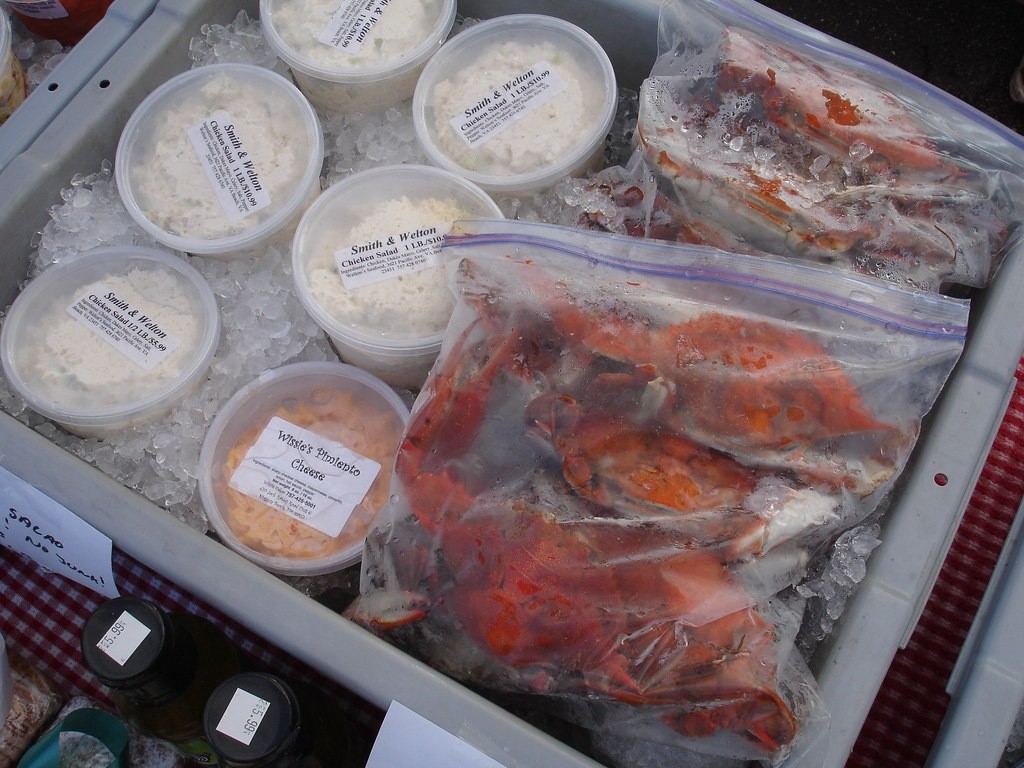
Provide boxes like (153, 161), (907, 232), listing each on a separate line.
(411, 14), (619, 195)
(198, 360), (411, 577)
(0, 245), (221, 441)
(114, 65), (324, 260)
(259, 0), (458, 119)
(290, 164), (507, 390)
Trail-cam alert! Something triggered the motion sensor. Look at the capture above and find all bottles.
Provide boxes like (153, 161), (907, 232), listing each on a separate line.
(80, 594), (242, 766)
(203, 671), (328, 767)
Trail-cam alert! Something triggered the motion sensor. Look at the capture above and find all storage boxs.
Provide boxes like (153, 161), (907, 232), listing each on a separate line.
(0, 0), (1024, 768)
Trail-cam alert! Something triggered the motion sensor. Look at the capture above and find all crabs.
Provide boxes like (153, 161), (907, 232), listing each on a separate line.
(341, 23), (1006, 753)
